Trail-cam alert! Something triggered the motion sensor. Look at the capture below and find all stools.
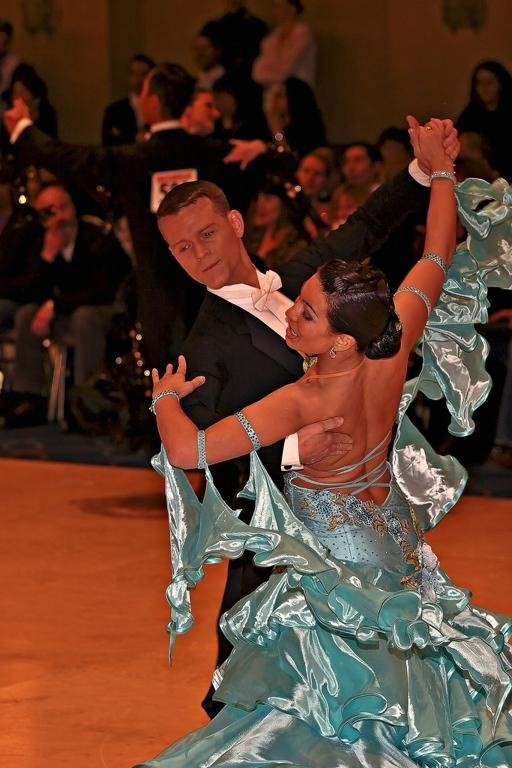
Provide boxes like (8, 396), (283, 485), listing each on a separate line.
(1, 326), (70, 428)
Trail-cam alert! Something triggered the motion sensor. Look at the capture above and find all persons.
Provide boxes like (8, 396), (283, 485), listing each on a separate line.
(1, 2), (512, 463)
(155, 114), (462, 721)
(149, 116), (511, 768)
